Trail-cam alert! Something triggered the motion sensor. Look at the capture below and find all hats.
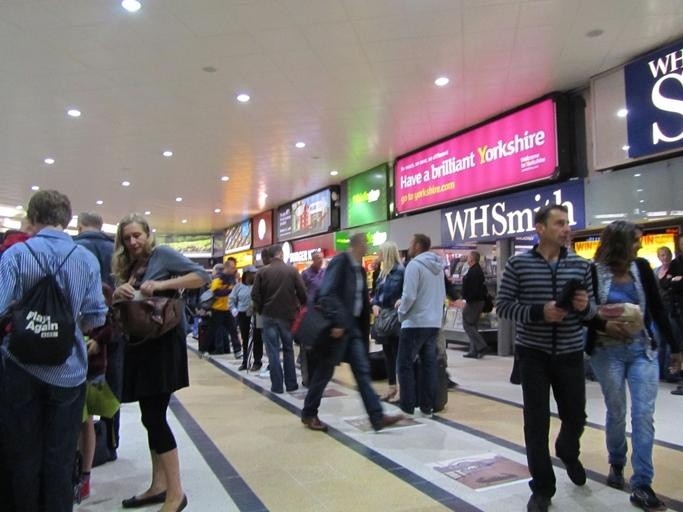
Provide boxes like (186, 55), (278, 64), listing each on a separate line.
(243, 264), (258, 273)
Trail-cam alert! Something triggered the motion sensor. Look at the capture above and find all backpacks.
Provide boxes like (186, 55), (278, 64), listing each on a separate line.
(9, 274), (76, 367)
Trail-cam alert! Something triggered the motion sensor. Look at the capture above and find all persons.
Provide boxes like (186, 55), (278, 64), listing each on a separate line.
(295, 249), (326, 370)
(1, 192), (40, 336)
(462, 250), (491, 359)
(251, 248), (273, 371)
(69, 237), (112, 502)
(111, 213), (213, 511)
(201, 256), (245, 360)
(661, 231), (683, 396)
(393, 232), (448, 414)
(590, 219), (666, 511)
(0, 214), (32, 240)
(228, 264), (259, 372)
(494, 203), (596, 512)
(1, 188), (110, 511)
(197, 263), (226, 353)
(251, 243), (309, 395)
(368, 258), (383, 316)
(399, 249), (460, 406)
(190, 280), (213, 340)
(301, 229), (405, 432)
(370, 241), (407, 404)
(73, 210), (121, 462)
(652, 245), (678, 384)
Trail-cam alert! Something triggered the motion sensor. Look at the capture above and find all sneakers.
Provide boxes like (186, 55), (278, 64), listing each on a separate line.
(239, 364), (247, 370)
(72, 479), (90, 500)
(251, 363), (262, 371)
(630, 486), (667, 512)
(271, 387), (283, 393)
(388, 391), (400, 403)
(260, 370), (270, 378)
(286, 384), (298, 391)
(260, 367), (270, 373)
(379, 387), (398, 402)
(607, 463), (625, 490)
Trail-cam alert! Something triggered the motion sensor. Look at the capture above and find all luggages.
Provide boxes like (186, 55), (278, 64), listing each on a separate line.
(413, 346), (449, 412)
(197, 319), (231, 353)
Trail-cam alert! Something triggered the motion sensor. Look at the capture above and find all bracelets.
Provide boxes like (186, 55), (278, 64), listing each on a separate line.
(112, 291), (116, 301)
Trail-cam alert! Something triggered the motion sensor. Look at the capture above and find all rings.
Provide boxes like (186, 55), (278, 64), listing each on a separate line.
(139, 282), (149, 290)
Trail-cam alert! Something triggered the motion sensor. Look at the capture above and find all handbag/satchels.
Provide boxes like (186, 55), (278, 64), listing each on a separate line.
(584, 328), (600, 355)
(109, 295), (184, 340)
(293, 307), (332, 349)
(482, 295), (494, 313)
(374, 308), (401, 338)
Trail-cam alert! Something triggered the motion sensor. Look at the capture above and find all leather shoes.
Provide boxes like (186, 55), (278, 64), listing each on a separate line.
(527, 489), (552, 512)
(677, 386), (683, 390)
(475, 346), (491, 358)
(373, 413), (403, 431)
(464, 351), (479, 358)
(671, 390), (683, 395)
(555, 442), (587, 486)
(176, 494), (188, 512)
(121, 490), (166, 507)
(302, 415), (328, 431)
